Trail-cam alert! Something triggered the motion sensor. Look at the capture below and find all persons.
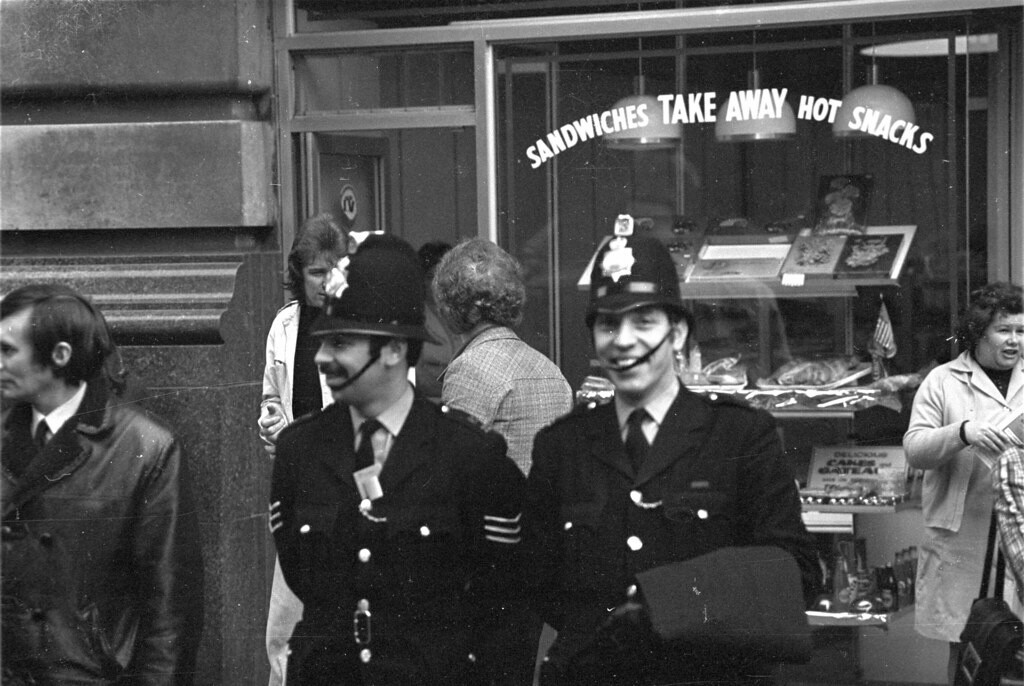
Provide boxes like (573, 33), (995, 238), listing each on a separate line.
(902, 282), (1023, 686)
(0, 282), (210, 686)
(678, 219), (796, 458)
(257, 211), (826, 686)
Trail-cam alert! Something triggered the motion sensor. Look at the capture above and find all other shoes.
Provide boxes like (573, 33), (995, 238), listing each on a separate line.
(755, 352), (873, 390)
(675, 353), (749, 392)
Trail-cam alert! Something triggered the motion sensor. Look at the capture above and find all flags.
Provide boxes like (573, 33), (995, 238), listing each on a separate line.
(873, 302), (897, 358)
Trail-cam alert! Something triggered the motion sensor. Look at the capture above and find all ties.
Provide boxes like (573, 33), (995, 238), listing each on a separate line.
(624, 408), (650, 475)
(352, 419), (382, 472)
(33, 421), (49, 452)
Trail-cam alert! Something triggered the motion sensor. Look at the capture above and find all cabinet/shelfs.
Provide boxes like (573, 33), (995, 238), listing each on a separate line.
(579, 276), (896, 628)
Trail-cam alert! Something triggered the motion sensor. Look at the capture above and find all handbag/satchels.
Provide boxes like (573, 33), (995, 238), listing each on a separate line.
(828, 541), (898, 615)
(953, 596), (1024, 686)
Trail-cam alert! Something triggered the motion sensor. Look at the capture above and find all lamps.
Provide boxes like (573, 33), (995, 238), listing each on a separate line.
(830, 21), (915, 139)
(716, 31), (796, 143)
(600, 1), (682, 149)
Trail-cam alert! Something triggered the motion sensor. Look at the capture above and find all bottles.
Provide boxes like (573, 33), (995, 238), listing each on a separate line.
(813, 537), (920, 612)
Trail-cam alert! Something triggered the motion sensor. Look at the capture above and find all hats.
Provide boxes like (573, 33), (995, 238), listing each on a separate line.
(306, 231), (443, 346)
(584, 235), (685, 319)
